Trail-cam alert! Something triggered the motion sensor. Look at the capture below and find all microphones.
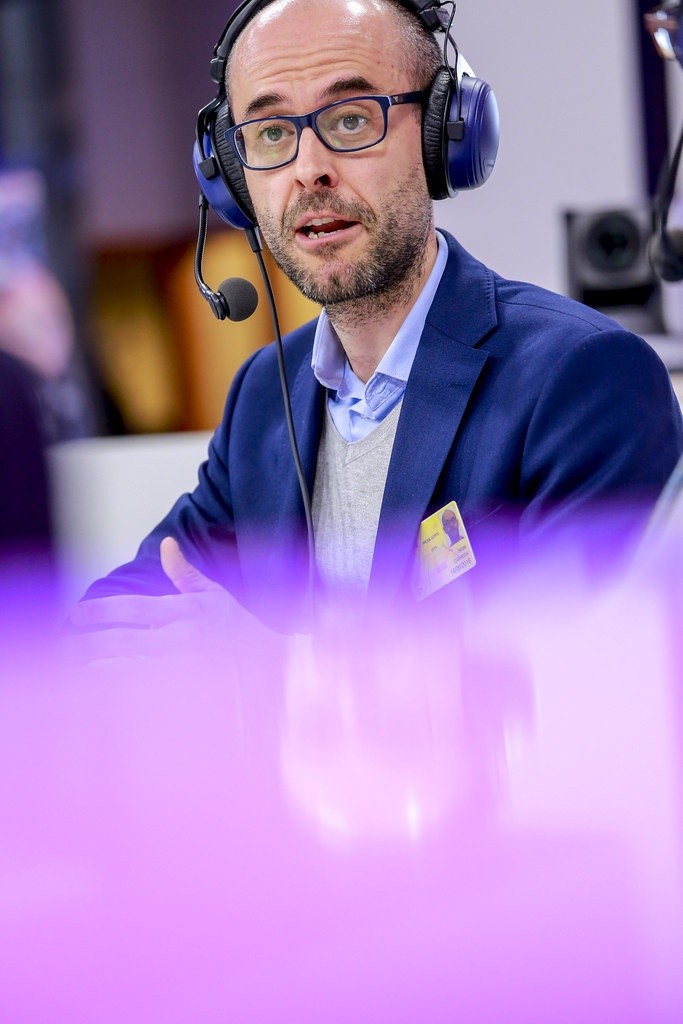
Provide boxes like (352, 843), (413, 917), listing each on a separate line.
(195, 205), (258, 322)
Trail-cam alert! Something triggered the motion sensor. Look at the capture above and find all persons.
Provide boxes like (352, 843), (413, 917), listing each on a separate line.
(54, 1), (683, 843)
(442, 509), (464, 547)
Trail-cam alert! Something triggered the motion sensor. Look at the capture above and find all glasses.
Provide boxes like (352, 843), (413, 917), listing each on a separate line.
(224, 92), (428, 170)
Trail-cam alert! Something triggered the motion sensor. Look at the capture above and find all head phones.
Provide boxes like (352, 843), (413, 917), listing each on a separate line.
(191, 0), (499, 230)
